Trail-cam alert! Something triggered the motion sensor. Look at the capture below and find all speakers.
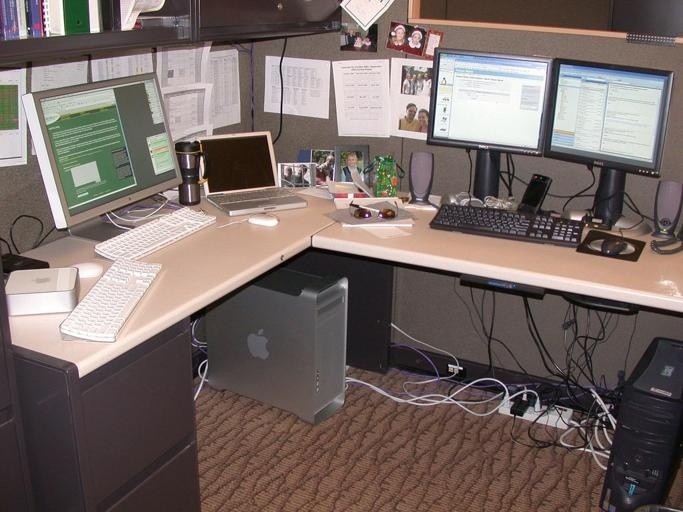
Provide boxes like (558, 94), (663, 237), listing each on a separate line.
(409, 152), (434, 205)
(651, 180), (683, 240)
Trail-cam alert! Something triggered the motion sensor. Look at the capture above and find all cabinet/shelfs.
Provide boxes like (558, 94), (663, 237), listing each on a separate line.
(0, 258), (34, 511)
(408, 0), (682, 44)
(0, 0), (194, 69)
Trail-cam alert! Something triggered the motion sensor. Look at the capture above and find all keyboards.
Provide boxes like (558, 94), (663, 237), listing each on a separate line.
(94, 205), (217, 265)
(59, 257), (163, 342)
(429, 203), (583, 249)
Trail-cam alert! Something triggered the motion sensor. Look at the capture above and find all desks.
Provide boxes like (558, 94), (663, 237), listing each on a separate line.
(4, 176), (394, 511)
(312, 189), (683, 512)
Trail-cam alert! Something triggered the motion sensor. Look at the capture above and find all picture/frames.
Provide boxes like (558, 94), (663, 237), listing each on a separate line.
(334, 145), (370, 186)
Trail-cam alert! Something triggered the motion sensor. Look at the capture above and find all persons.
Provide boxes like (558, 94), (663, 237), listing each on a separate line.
(285, 165), (308, 187)
(400, 71), (432, 95)
(407, 28), (422, 57)
(399, 102), (421, 131)
(340, 152), (362, 182)
(418, 108), (429, 133)
(346, 30), (372, 49)
(317, 154), (333, 181)
(388, 25), (407, 51)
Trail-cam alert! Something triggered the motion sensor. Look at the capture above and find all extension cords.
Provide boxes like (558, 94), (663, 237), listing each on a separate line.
(498, 398), (573, 429)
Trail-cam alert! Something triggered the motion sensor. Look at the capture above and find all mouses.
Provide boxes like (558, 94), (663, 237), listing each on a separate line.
(248, 214), (279, 226)
(602, 237), (627, 255)
(74, 263), (105, 279)
(378, 209), (397, 219)
(352, 207), (371, 220)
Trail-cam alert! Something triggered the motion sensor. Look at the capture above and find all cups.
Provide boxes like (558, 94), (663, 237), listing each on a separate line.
(175, 141), (209, 205)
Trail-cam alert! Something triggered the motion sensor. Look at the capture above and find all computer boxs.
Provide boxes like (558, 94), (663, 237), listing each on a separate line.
(597, 336), (683, 512)
(206, 266), (348, 423)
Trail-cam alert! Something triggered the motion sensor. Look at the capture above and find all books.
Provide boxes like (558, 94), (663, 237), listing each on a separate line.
(1, 0), (45, 41)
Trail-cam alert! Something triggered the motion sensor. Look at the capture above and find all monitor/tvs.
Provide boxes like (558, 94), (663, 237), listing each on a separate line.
(20, 73), (185, 242)
(544, 56), (674, 235)
(427, 48), (552, 202)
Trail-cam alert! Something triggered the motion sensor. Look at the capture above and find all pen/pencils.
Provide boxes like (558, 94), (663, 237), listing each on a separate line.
(350, 204), (380, 213)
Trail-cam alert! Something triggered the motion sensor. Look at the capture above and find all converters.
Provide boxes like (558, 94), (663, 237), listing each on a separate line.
(0, 251), (48, 275)
(511, 400), (529, 418)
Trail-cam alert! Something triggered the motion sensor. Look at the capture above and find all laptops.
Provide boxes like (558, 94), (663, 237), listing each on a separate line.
(197, 130), (307, 215)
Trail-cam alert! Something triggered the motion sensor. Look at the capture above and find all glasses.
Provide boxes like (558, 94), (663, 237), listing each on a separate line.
(349, 200), (398, 219)
